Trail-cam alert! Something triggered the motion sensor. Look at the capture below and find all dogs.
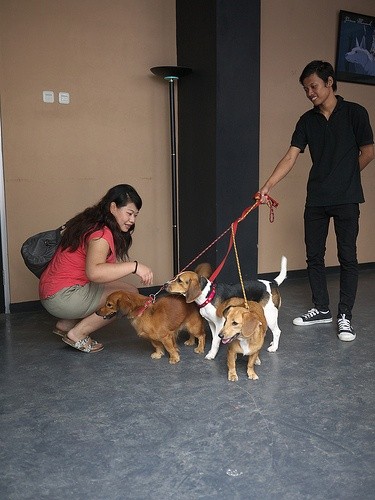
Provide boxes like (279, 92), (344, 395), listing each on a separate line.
(216, 297), (268, 383)
(163, 254), (290, 360)
(95, 261), (214, 365)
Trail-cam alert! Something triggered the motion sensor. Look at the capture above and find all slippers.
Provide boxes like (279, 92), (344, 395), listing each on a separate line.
(52, 326), (106, 353)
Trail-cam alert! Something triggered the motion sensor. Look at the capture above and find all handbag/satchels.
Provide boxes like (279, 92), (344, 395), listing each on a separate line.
(20, 221), (67, 279)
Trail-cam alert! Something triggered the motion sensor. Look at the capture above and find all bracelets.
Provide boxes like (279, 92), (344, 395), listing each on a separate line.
(132, 261), (138, 274)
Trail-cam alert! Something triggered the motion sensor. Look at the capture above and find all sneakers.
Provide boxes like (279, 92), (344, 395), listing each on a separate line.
(336, 314), (357, 342)
(292, 308), (333, 326)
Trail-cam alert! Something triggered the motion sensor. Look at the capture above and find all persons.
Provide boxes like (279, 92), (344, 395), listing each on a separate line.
(256, 60), (375, 341)
(38, 184), (154, 354)
